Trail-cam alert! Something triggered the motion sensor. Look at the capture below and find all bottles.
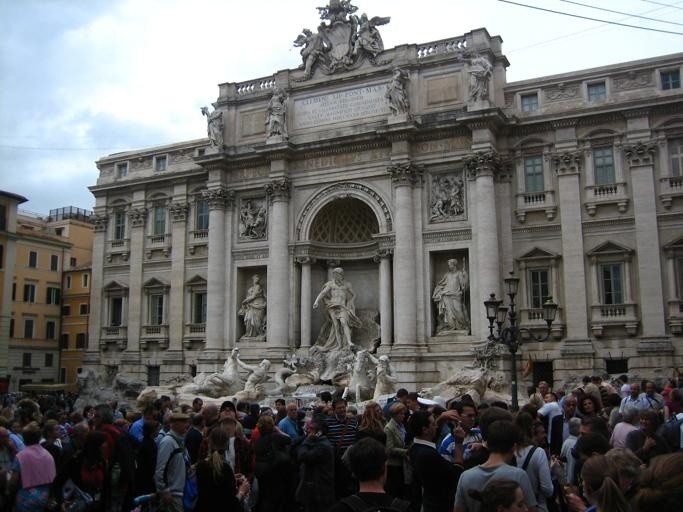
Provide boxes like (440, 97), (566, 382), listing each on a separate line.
(110, 461), (121, 484)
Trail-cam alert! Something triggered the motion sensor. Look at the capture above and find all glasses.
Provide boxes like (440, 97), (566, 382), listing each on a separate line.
(462, 414), (475, 417)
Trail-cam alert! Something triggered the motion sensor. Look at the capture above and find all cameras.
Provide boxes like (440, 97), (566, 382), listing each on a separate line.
(455, 421), (461, 428)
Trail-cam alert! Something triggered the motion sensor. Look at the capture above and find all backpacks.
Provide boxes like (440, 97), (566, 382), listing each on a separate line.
(665, 414), (683, 452)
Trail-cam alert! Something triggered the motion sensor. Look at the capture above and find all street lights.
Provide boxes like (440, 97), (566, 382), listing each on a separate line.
(484, 271), (559, 413)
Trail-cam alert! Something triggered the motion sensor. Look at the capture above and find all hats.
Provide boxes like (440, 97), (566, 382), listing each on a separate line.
(172, 413), (190, 420)
(219, 416), (237, 425)
(219, 401), (237, 414)
(416, 396), (438, 410)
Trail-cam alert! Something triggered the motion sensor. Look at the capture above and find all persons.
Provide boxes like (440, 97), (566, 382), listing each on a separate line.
(432, 256), (471, 331)
(428, 174), (464, 221)
(290, 28), (324, 82)
(240, 201), (266, 239)
(238, 274), (266, 337)
(313, 268), (356, 346)
(457, 52), (493, 100)
(352, 12), (391, 58)
(237, 346), (275, 403)
(384, 67), (410, 115)
(264, 84), (288, 139)
(367, 352), (396, 398)
(199, 103), (224, 147)
